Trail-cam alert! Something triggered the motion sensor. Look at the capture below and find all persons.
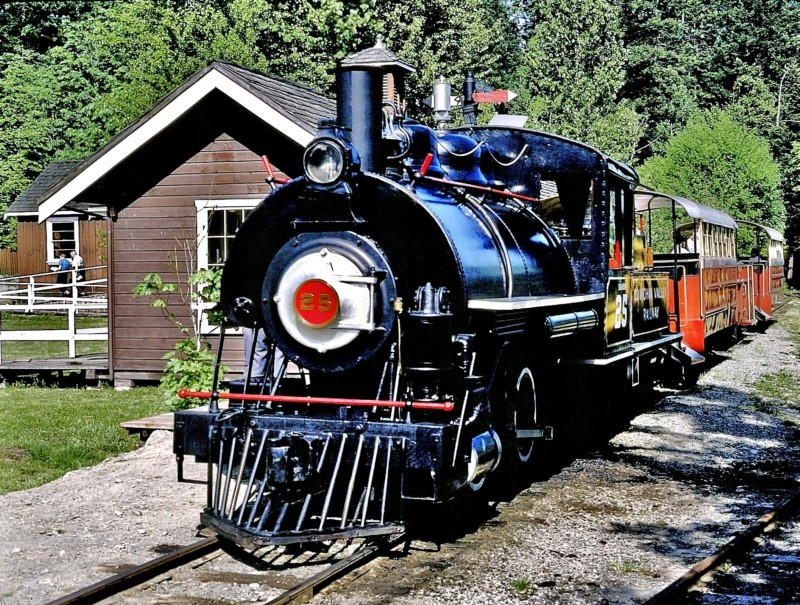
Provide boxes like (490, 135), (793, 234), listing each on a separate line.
(56, 251), (86, 297)
(671, 233), (695, 254)
(787, 251), (794, 289)
(749, 248), (761, 264)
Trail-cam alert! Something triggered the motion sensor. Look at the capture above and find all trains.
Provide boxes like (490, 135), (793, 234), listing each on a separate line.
(173, 36), (789, 547)
